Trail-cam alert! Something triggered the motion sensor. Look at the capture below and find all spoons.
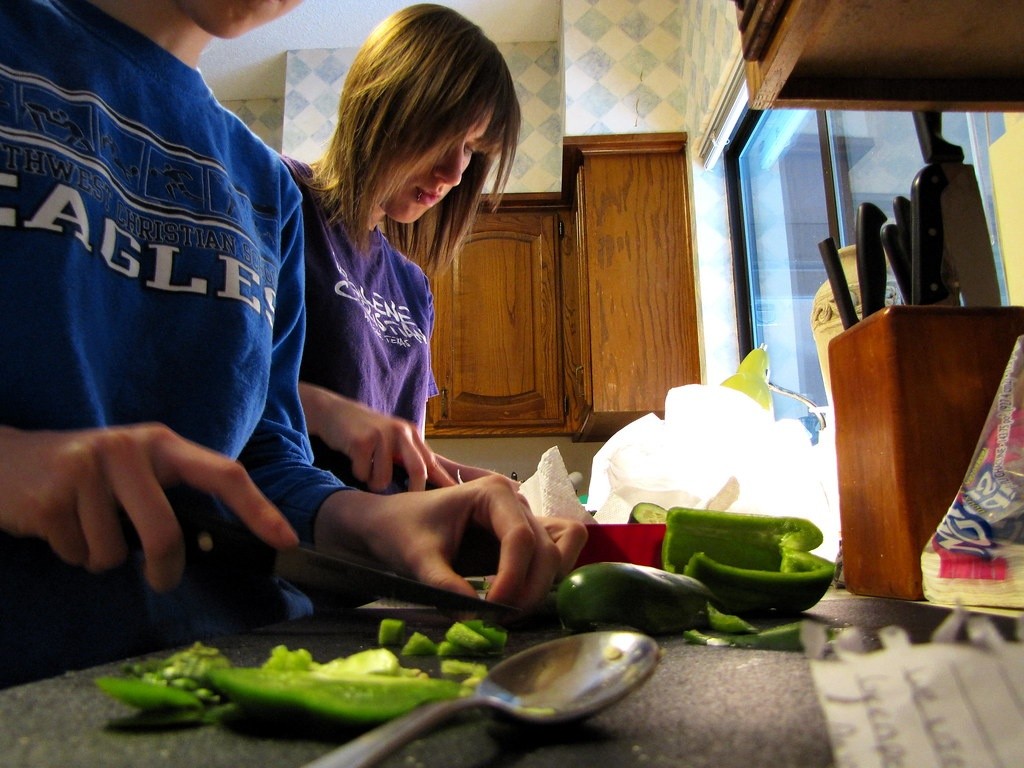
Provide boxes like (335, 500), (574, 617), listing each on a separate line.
(292, 631), (662, 767)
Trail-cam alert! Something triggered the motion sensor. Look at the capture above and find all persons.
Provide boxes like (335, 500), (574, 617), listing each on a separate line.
(0, 0), (589, 691)
(282, 3), (520, 495)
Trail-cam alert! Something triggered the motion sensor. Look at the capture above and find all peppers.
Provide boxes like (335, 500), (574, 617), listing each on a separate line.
(90, 505), (837, 720)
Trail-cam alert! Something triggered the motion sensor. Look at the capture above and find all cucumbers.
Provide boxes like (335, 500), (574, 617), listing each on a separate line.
(627, 502), (669, 523)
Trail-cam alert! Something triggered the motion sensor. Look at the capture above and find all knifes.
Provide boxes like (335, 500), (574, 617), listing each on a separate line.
(110, 495), (525, 628)
(915, 112), (1002, 311)
(816, 168), (958, 332)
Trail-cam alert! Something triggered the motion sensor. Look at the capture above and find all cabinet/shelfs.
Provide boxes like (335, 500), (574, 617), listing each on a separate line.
(734, 0), (1024, 111)
(412, 131), (691, 440)
